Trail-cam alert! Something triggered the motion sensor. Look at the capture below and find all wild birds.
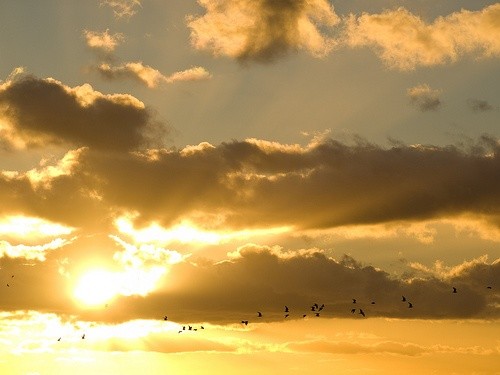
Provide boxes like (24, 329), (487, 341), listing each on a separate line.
(311, 303), (325, 317)
(81, 334), (85, 339)
(58, 337), (61, 341)
(178, 325), (204, 333)
(402, 295), (413, 308)
(452, 287), (457, 294)
(241, 320), (248, 325)
(257, 312), (262, 317)
(164, 316), (168, 320)
(284, 306), (289, 312)
(487, 286), (492, 289)
(303, 314), (306, 317)
(350, 299), (375, 317)
(284, 314), (289, 318)
(6, 274), (14, 287)
